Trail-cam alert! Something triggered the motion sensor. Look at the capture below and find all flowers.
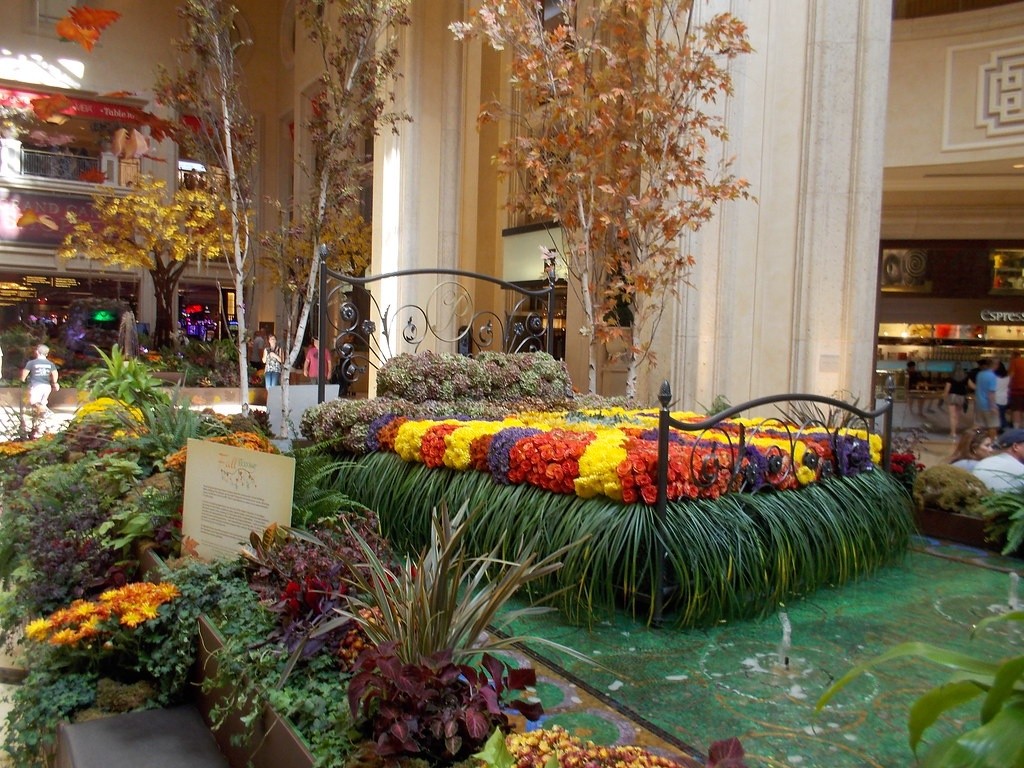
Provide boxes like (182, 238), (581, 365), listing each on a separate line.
(366, 402), (921, 506)
(22, 574), (182, 659)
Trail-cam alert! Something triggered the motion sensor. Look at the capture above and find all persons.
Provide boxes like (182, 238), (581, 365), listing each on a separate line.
(902, 350), (1024, 495)
(248, 328), (332, 392)
(20, 344), (60, 417)
